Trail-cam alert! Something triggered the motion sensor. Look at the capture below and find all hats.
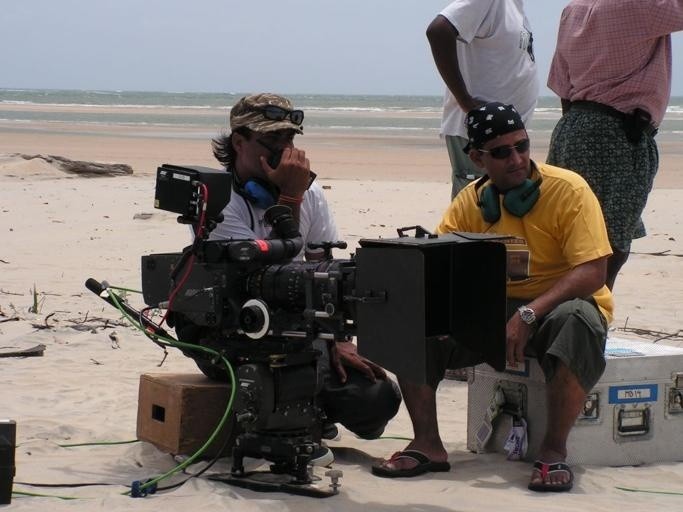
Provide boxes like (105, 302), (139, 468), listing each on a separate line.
(229, 92), (304, 137)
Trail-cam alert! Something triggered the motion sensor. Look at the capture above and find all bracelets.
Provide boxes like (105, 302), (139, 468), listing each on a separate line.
(276, 194), (302, 210)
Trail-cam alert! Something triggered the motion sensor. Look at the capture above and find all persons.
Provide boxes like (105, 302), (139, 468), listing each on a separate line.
(176, 92), (402, 469)
(373, 103), (614, 493)
(426, 0), (538, 203)
(547, 1), (683, 292)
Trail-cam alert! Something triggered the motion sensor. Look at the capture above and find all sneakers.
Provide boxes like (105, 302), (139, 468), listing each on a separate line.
(307, 445), (335, 467)
(322, 424), (341, 442)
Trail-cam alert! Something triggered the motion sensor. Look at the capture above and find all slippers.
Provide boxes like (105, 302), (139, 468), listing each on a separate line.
(371, 448), (452, 478)
(527, 455), (574, 494)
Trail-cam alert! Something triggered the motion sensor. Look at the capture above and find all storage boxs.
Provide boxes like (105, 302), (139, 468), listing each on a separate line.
(467, 333), (683, 469)
(136, 373), (244, 459)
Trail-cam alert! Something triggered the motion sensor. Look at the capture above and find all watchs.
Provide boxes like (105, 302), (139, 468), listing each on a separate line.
(517, 304), (538, 332)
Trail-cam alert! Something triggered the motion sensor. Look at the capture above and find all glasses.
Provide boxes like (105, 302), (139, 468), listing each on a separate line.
(479, 137), (530, 160)
(252, 105), (304, 126)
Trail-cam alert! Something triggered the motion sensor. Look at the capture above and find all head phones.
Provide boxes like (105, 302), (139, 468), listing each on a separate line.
(232, 157), (281, 209)
(474, 157), (543, 224)
